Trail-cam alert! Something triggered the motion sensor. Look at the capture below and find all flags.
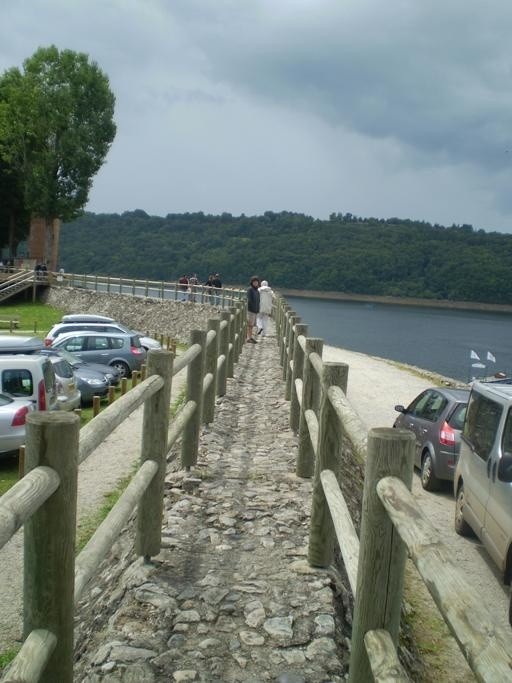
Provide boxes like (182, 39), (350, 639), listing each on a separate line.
(470, 349), (480, 360)
(487, 350), (495, 362)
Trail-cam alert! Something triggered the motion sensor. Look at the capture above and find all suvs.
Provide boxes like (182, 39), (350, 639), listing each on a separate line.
(0, 313), (163, 454)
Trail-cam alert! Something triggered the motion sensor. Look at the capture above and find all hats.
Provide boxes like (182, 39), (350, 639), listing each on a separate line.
(261, 280), (268, 286)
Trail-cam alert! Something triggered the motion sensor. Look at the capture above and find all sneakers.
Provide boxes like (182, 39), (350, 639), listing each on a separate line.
(247, 338), (257, 344)
(257, 329), (263, 334)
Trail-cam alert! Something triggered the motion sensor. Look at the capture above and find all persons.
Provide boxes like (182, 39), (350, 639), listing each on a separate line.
(189, 271), (199, 302)
(247, 276), (260, 343)
(257, 279), (276, 336)
(179, 273), (189, 300)
(203, 273), (215, 304)
(212, 273), (222, 305)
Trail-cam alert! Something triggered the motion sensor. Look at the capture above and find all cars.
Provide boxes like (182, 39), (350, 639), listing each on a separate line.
(394, 385), (471, 492)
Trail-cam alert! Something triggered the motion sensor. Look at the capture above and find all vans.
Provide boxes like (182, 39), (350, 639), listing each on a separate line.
(454, 372), (509, 586)
